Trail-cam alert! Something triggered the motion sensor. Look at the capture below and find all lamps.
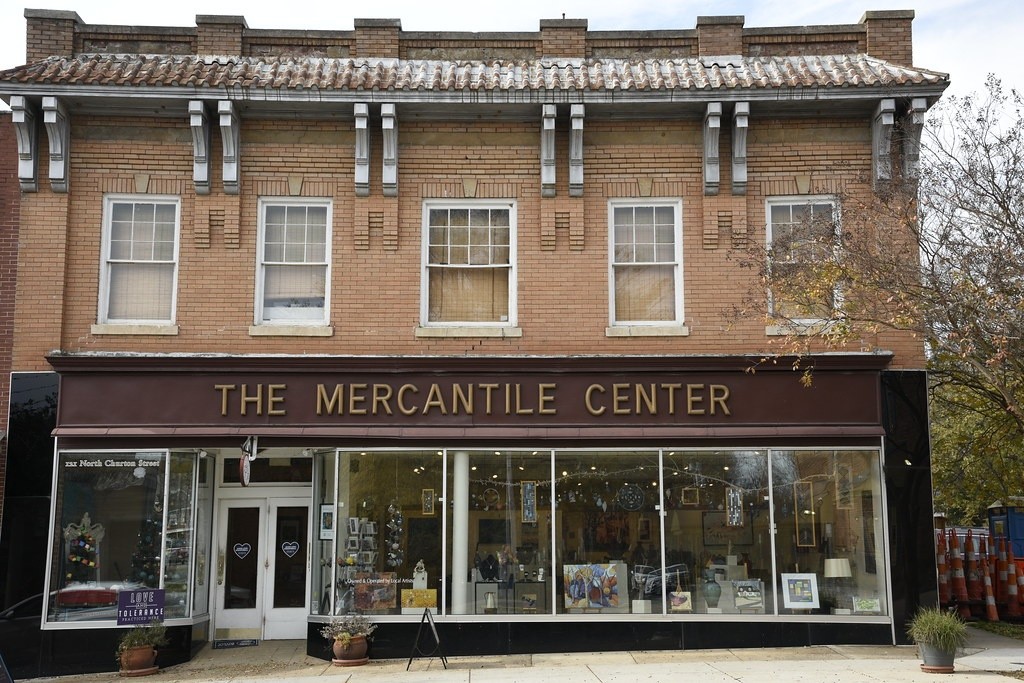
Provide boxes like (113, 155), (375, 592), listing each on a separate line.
(823, 559), (853, 614)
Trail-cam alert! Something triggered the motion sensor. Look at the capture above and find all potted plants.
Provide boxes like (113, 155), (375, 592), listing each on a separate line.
(908, 605), (968, 673)
(113, 622), (169, 677)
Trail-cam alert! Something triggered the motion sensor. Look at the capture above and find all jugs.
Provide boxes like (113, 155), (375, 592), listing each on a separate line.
(484, 592), (496, 608)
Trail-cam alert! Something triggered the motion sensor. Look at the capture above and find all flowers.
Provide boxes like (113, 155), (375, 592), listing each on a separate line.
(319, 614), (379, 642)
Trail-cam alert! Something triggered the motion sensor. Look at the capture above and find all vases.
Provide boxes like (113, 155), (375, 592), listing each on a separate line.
(332, 636), (370, 666)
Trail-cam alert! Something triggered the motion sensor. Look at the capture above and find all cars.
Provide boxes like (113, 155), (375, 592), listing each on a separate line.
(0, 579), (151, 666)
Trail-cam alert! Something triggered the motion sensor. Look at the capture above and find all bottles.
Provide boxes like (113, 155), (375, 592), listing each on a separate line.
(702, 570), (721, 607)
